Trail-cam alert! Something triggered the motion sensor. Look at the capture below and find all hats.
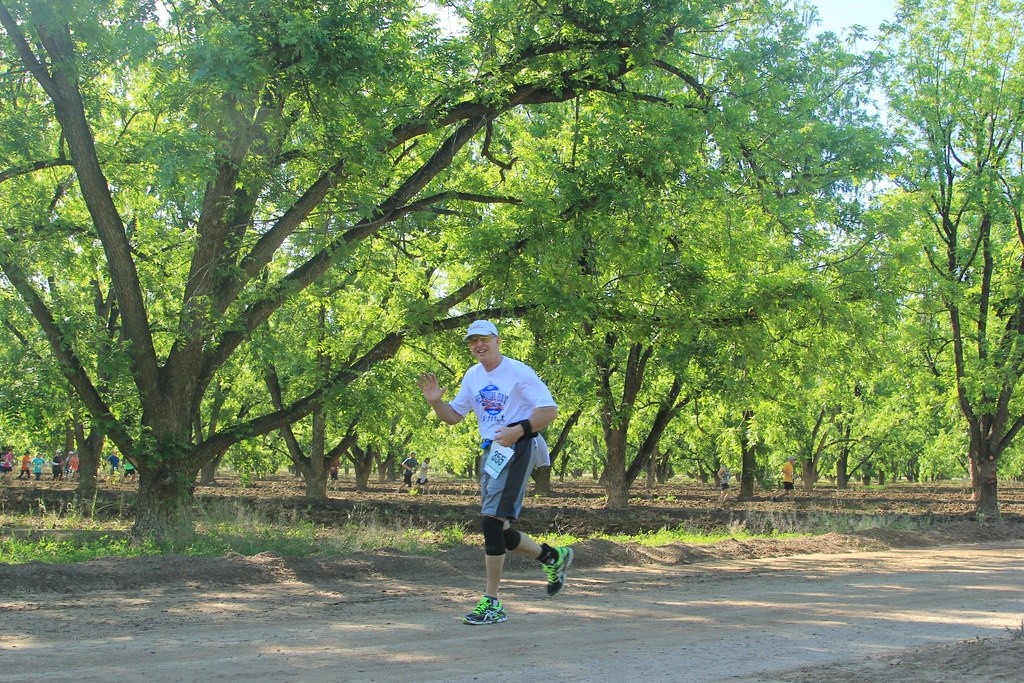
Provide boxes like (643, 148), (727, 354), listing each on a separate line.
(463, 320), (498, 340)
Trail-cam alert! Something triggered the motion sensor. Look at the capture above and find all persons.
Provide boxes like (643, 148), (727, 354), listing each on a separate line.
(718, 462), (737, 504)
(415, 319), (575, 625)
(1, 449), (136, 484)
(330, 456), (340, 486)
(776, 455), (798, 502)
(397, 452), (431, 494)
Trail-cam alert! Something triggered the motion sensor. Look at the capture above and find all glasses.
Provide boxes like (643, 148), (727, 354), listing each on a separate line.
(468, 336), (495, 346)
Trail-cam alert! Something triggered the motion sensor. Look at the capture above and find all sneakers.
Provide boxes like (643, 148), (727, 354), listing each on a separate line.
(463, 597), (507, 624)
(542, 546), (574, 597)
